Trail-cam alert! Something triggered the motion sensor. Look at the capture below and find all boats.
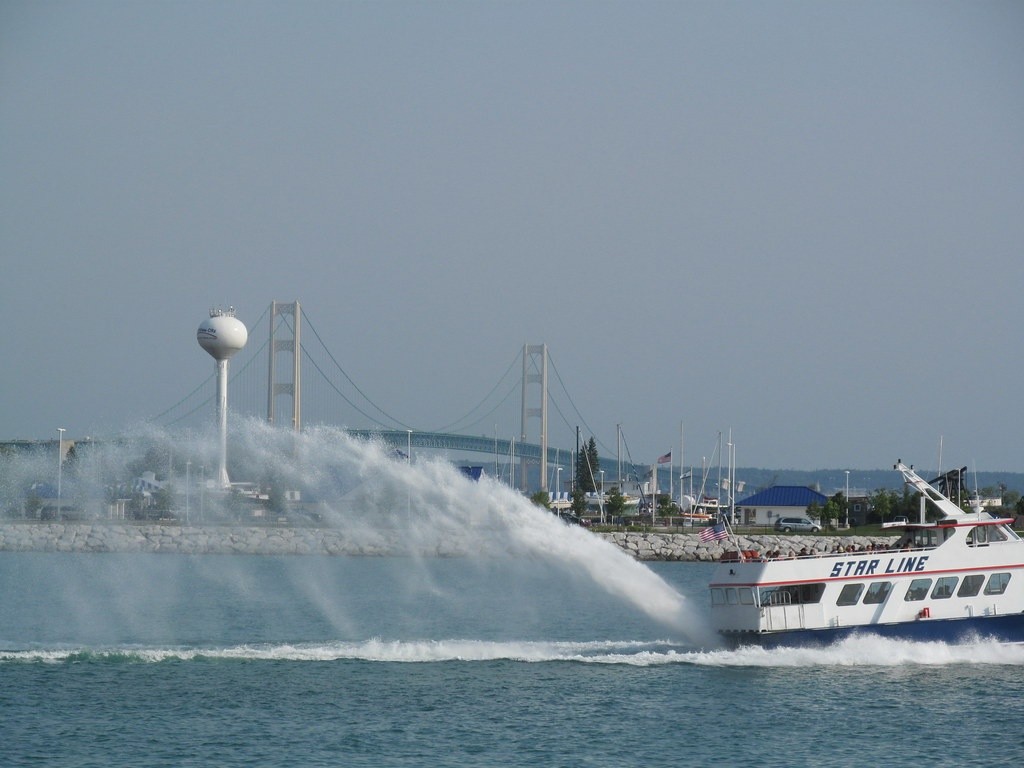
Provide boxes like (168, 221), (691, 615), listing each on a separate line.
(708, 436), (1024, 649)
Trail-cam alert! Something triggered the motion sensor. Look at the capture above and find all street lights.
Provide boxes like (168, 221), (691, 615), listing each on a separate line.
(556, 467), (563, 518)
(844, 470), (850, 529)
(406, 429), (413, 516)
(56, 427), (66, 523)
(725, 442), (737, 524)
(600, 470), (605, 523)
(182, 461), (193, 527)
(197, 465), (205, 521)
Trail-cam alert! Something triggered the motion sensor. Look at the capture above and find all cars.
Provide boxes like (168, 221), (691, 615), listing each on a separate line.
(555, 512), (592, 526)
(774, 516), (823, 533)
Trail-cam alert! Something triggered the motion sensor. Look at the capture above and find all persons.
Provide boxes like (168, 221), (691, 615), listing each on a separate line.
(788, 548), (816, 560)
(864, 588), (888, 604)
(831, 542), (890, 556)
(721, 549), (780, 563)
(958, 585), (979, 596)
(906, 588), (927, 601)
(1001, 583), (1007, 593)
(896, 538), (923, 552)
(581, 518), (592, 530)
(937, 586), (949, 595)
(793, 590), (799, 604)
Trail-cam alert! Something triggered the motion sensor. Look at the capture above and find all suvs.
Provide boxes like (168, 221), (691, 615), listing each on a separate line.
(39, 504), (101, 522)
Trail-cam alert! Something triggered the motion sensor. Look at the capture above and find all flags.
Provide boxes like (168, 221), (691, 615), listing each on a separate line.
(658, 452), (671, 464)
(699, 521), (729, 544)
